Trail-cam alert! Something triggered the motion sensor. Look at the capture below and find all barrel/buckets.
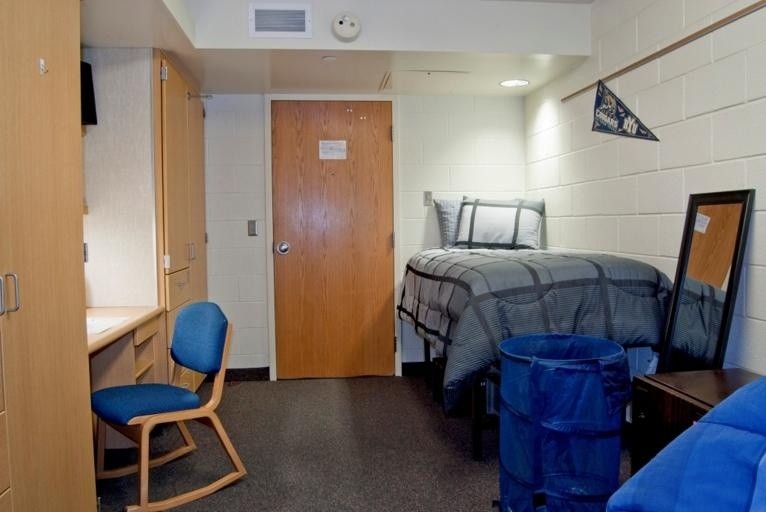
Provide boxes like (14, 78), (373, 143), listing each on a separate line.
(497, 330), (630, 511)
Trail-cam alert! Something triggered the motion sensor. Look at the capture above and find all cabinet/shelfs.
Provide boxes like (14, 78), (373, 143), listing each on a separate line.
(78, 45), (208, 394)
(629, 366), (762, 480)
(0, 0), (101, 511)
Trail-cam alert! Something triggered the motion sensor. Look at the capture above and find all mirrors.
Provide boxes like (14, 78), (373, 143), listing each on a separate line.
(654, 187), (756, 372)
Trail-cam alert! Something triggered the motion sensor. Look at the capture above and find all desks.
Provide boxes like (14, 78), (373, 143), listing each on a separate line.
(84, 305), (164, 452)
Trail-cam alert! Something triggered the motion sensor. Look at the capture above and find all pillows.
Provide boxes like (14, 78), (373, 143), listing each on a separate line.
(433, 193), (545, 252)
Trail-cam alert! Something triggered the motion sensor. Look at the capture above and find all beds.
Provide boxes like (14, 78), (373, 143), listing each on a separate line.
(408, 248), (668, 461)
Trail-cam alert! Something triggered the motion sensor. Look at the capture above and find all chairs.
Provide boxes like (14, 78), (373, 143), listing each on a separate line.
(88, 301), (250, 510)
